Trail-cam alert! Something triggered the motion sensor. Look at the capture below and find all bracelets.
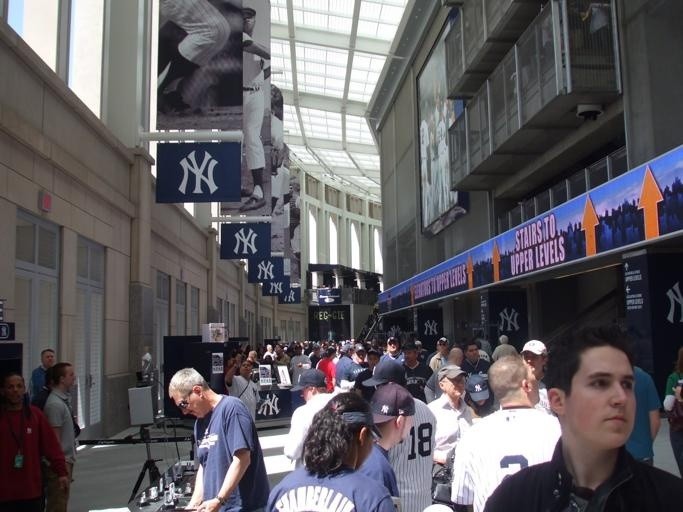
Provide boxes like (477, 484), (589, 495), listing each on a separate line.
(215, 495), (226, 507)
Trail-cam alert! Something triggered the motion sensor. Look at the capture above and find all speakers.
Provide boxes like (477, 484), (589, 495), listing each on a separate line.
(126, 384), (159, 427)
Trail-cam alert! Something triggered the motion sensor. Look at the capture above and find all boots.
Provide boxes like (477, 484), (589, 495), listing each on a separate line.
(157, 48), (201, 115)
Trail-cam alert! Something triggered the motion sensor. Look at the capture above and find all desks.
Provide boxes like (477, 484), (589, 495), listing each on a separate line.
(126, 460), (201, 512)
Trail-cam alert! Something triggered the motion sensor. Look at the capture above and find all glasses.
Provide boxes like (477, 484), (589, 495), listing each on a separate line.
(437, 343), (445, 346)
(176, 384), (203, 409)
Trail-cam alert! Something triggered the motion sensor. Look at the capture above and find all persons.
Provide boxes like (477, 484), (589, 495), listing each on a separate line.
(483, 321), (683, 512)
(358, 305), (465, 404)
(465, 374), (491, 419)
(141, 347), (152, 371)
(418, 78), (456, 229)
(491, 335), (519, 361)
(260, 330), (358, 388)
(426, 363), (472, 511)
(352, 381), (416, 511)
(361, 359), (437, 512)
(157, 1), (231, 114)
(42, 362), (76, 512)
(283, 368), (339, 471)
(499, 0), (616, 97)
(465, 329), (492, 381)
(673, 347), (682, 403)
(224, 359), (260, 423)
(450, 355), (563, 512)
(169, 368), (270, 512)
(662, 370), (683, 481)
(238, 6), (269, 211)
(520, 339), (569, 417)
(0, 370), (69, 511)
(28, 348), (56, 402)
(29, 367), (56, 414)
(603, 323), (661, 469)
(265, 392), (397, 512)
(224, 343), (260, 375)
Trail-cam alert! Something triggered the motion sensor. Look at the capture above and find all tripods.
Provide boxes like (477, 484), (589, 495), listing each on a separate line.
(123, 429), (164, 504)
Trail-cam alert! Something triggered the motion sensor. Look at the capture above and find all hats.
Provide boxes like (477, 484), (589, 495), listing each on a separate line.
(368, 382), (415, 424)
(387, 336), (398, 344)
(362, 359), (407, 387)
(437, 364), (467, 382)
(342, 412), (382, 441)
(464, 375), (489, 402)
(519, 340), (547, 356)
(400, 342), (418, 351)
(356, 345), (365, 352)
(340, 344), (350, 352)
(289, 368), (326, 392)
(438, 337), (449, 345)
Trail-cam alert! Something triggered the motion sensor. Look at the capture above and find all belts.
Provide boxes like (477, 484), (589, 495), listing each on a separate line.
(243, 86), (261, 92)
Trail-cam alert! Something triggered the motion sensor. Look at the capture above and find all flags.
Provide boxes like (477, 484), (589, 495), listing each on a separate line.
(318, 289), (342, 305)
(248, 256), (284, 283)
(155, 140), (242, 204)
(219, 222), (272, 260)
(278, 287), (300, 304)
(262, 275), (290, 296)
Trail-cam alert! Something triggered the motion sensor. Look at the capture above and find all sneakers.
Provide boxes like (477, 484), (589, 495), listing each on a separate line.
(241, 185), (253, 195)
(240, 194), (266, 211)
